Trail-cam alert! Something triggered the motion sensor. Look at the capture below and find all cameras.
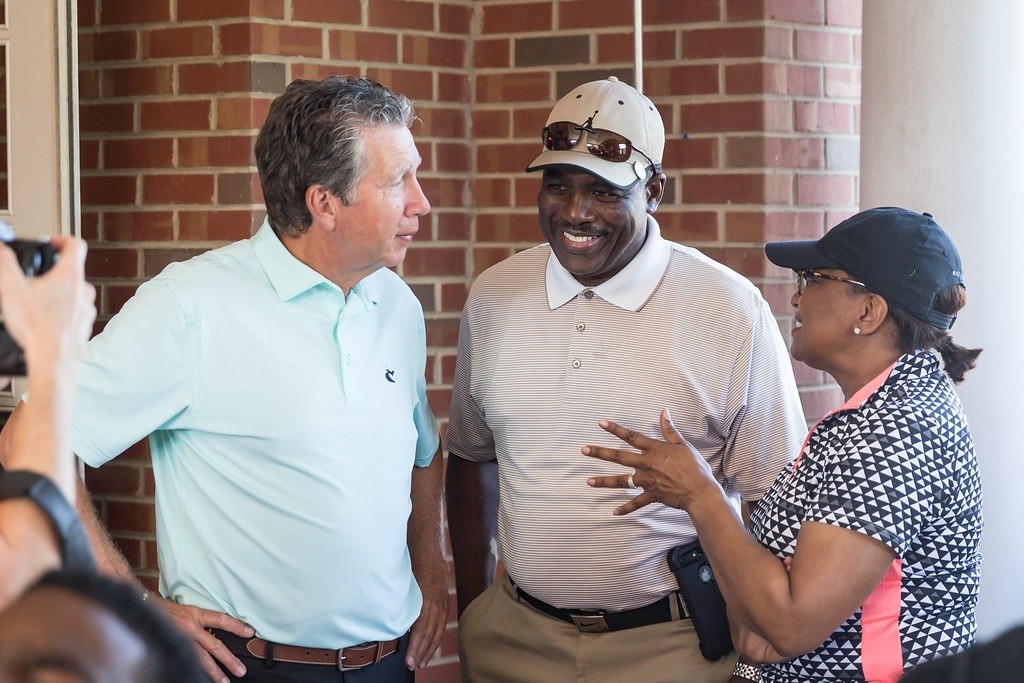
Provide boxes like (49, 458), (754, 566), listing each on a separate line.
(0, 239), (54, 377)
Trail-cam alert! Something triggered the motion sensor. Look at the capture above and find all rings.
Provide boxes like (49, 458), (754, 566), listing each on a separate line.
(628, 472), (638, 489)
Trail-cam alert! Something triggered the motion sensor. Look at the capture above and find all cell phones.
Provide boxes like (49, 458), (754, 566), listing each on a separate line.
(667, 539), (734, 662)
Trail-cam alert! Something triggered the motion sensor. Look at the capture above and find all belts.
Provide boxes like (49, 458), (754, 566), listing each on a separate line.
(206, 628), (398, 671)
(507, 574), (690, 634)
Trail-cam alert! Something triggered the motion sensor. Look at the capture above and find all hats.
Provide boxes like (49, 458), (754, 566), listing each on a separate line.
(525, 76), (665, 190)
(765, 207), (967, 328)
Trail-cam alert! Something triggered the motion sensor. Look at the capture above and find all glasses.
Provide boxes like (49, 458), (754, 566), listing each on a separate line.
(542, 121), (656, 176)
(796, 271), (865, 296)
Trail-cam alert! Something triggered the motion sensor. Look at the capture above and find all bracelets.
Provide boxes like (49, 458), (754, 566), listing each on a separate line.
(141, 589), (154, 601)
(733, 662), (765, 683)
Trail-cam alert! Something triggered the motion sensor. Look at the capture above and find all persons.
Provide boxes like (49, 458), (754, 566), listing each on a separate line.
(581, 207), (985, 683)
(0, 235), (209, 683)
(73, 75), (450, 683)
(445, 76), (809, 683)
